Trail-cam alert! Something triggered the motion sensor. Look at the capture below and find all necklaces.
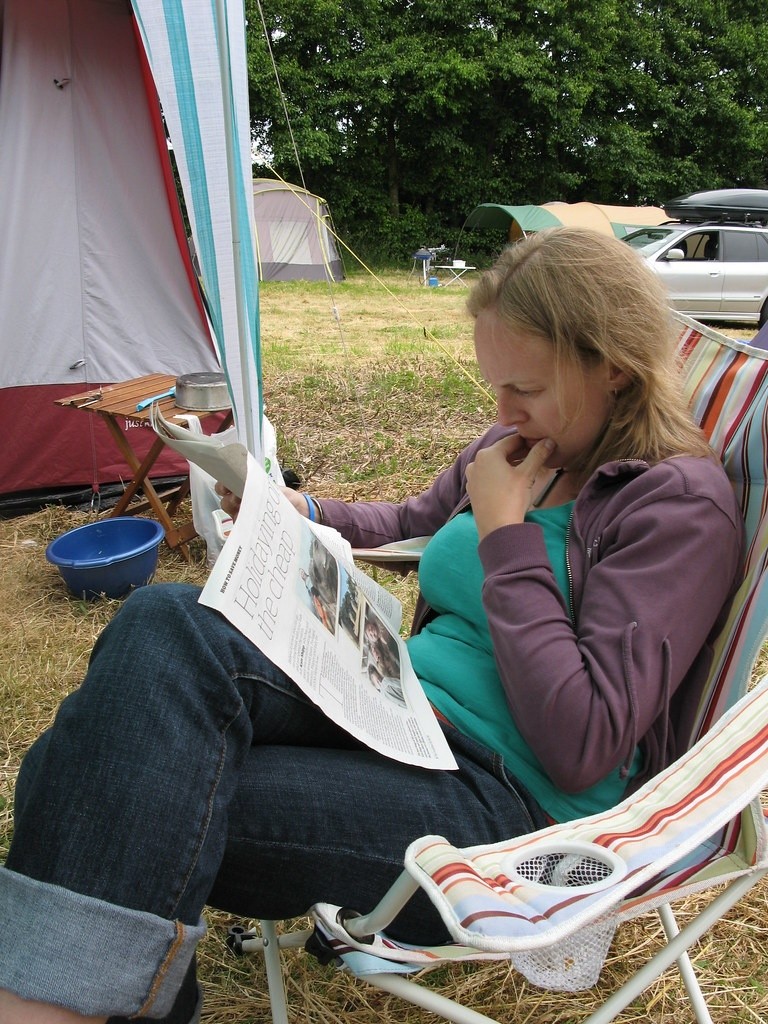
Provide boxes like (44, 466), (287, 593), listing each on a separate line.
(533, 467), (564, 508)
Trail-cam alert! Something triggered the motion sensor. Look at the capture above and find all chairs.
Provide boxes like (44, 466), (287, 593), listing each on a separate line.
(675, 239), (688, 257)
(224, 306), (768, 1024)
(704, 234), (719, 261)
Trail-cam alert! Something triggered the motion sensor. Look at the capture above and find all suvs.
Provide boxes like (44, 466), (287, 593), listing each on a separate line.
(619, 187), (768, 333)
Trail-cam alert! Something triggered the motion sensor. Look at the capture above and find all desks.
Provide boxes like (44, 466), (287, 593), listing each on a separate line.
(55, 373), (233, 563)
(435, 266), (477, 287)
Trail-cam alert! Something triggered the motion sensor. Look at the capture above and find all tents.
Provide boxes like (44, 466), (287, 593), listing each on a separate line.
(456, 201), (676, 261)
(251, 179), (346, 282)
(0, 0), (262, 519)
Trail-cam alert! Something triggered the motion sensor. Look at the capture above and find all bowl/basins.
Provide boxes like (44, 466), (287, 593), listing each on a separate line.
(46, 516), (165, 601)
(174, 372), (233, 411)
(453, 260), (466, 267)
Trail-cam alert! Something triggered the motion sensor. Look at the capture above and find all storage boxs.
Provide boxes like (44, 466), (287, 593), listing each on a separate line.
(452, 260), (466, 267)
(429, 278), (438, 287)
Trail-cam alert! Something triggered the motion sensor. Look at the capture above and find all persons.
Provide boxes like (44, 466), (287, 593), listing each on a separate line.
(310, 587), (403, 706)
(0, 227), (744, 1024)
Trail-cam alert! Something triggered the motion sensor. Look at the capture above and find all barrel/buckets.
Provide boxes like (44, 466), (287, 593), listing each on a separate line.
(429, 278), (438, 287)
